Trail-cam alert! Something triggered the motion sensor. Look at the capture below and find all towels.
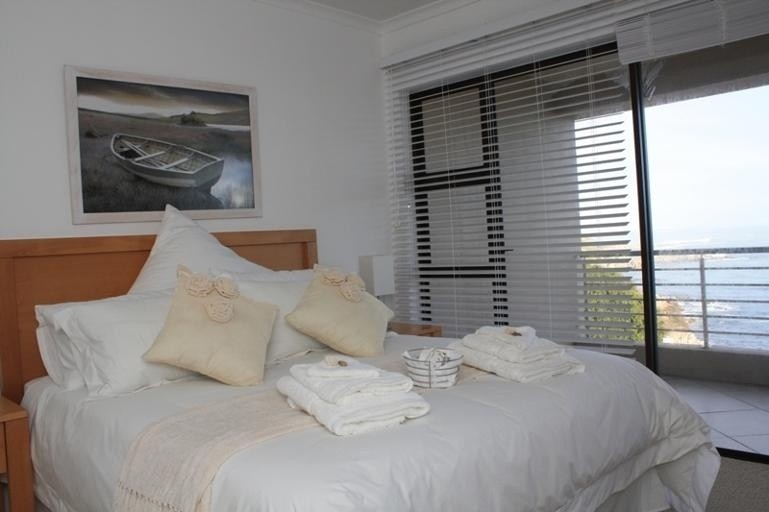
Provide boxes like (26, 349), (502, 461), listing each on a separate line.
(462, 331), (575, 362)
(306, 355), (383, 378)
(474, 325), (537, 350)
(444, 342), (584, 384)
(290, 357), (413, 404)
(276, 376), (429, 434)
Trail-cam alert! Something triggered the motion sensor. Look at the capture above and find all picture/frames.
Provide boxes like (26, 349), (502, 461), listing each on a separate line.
(61, 62), (265, 224)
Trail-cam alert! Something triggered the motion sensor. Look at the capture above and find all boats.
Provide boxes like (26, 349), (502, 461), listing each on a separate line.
(108, 131), (227, 192)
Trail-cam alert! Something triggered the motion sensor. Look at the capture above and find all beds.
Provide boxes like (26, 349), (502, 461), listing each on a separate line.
(0, 229), (721, 512)
